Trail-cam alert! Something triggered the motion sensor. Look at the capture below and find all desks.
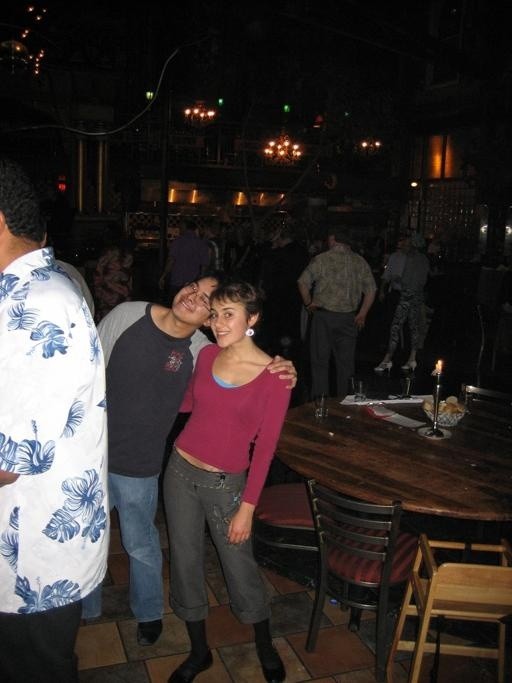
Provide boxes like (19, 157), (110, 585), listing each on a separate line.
(276, 393), (512, 530)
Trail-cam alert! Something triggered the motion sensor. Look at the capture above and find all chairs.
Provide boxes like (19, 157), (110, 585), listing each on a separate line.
(462, 384), (512, 402)
(304, 479), (423, 681)
(253, 484), (350, 609)
(384, 532), (511, 681)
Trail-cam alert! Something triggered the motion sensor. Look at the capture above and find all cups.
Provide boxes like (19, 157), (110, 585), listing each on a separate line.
(311, 389), (330, 418)
(349, 374), (369, 402)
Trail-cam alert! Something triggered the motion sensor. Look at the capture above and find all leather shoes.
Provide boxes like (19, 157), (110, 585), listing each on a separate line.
(137, 619), (163, 646)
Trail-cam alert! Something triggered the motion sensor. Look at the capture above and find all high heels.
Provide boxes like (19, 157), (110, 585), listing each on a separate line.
(374, 361), (392, 372)
(402, 360), (417, 371)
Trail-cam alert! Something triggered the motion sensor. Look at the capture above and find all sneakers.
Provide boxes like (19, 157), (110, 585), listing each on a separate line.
(167, 645), (213, 682)
(254, 640), (286, 683)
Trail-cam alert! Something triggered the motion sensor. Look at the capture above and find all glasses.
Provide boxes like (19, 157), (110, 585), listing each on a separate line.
(184, 281), (211, 313)
